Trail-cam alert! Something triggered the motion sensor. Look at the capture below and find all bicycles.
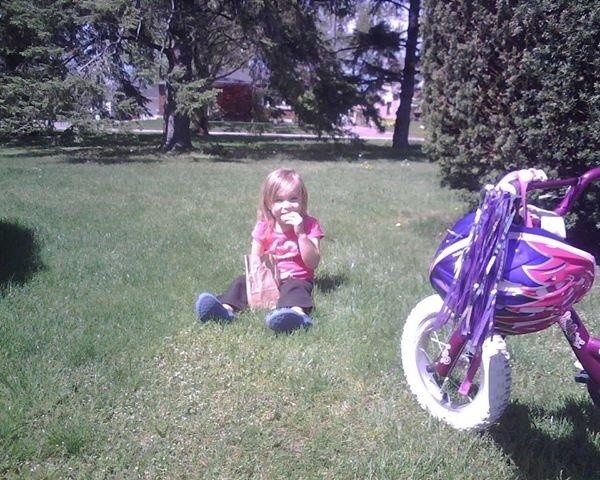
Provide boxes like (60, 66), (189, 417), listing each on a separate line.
(401, 167), (599, 434)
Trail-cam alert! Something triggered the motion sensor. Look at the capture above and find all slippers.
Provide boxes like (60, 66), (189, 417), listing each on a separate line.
(197, 294), (235, 325)
(265, 308), (314, 332)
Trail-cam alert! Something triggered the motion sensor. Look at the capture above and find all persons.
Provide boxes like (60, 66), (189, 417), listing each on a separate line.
(196, 167), (324, 333)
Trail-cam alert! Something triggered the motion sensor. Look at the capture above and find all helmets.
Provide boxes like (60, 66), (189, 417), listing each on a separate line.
(430, 212), (596, 336)
